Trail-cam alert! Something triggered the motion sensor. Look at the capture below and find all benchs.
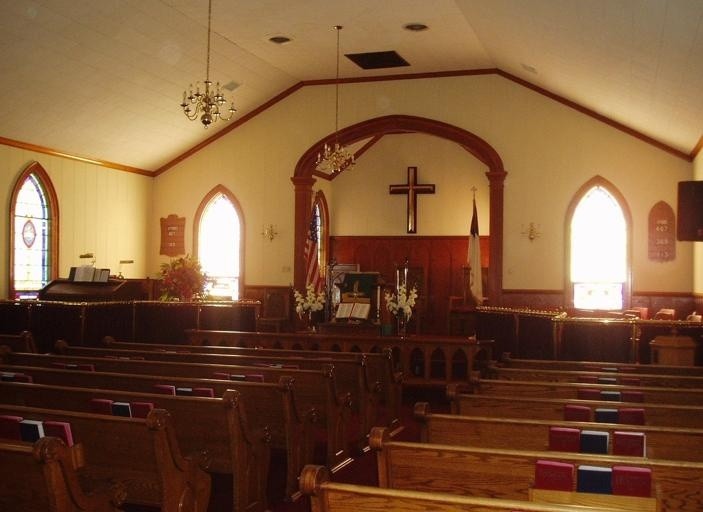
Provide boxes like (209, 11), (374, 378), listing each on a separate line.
(0, 299), (703, 512)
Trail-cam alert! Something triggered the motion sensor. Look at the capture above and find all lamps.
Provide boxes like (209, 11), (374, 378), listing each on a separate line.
(314, 24), (356, 175)
(178, 0), (239, 128)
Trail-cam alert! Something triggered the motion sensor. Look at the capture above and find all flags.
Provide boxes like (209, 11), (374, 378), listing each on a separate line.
(465, 197), (483, 304)
(303, 201), (324, 295)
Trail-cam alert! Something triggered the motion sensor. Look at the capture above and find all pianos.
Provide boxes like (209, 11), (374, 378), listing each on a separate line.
(38, 277), (146, 301)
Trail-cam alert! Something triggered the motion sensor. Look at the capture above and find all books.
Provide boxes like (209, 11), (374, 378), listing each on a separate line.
(530, 362), (653, 499)
(335, 303), (370, 321)
(73, 266), (109, 282)
(0, 349), (299, 446)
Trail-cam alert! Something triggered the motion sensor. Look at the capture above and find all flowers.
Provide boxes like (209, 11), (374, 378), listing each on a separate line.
(288, 269), (323, 318)
(161, 253), (210, 300)
(380, 278), (427, 318)
